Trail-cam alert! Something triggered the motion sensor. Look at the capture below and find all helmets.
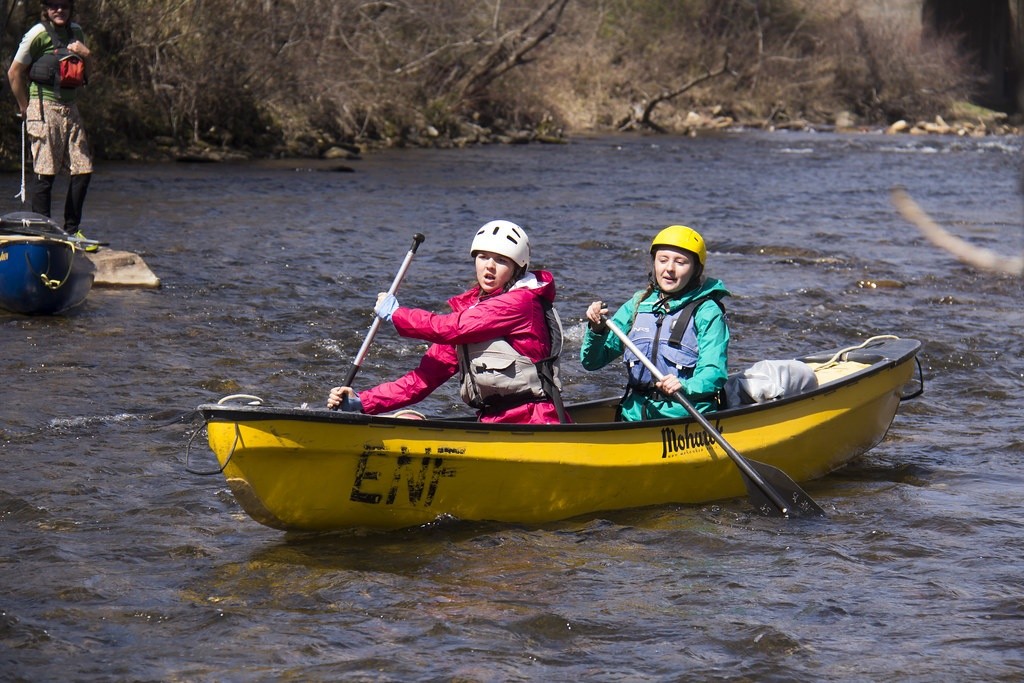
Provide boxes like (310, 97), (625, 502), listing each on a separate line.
(469, 219), (531, 276)
(649, 225), (707, 282)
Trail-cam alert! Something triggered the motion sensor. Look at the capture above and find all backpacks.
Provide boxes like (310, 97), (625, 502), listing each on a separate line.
(29, 21), (88, 101)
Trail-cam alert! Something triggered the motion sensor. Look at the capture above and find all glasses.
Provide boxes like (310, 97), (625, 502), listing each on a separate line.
(48, 3), (70, 10)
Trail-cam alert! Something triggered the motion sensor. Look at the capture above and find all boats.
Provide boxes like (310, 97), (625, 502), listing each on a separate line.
(0, 211), (96, 316)
(195, 334), (927, 540)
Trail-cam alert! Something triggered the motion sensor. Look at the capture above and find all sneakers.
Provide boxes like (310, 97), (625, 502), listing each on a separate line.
(66, 230), (102, 253)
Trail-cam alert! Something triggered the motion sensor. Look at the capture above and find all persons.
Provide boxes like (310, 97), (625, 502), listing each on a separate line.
(8, 0), (98, 251)
(327, 220), (574, 425)
(580, 225), (731, 423)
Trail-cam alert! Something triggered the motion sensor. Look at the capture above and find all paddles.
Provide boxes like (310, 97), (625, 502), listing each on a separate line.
(4, 226), (110, 246)
(328, 233), (426, 412)
(598, 302), (827, 520)
(15, 112), (31, 211)
